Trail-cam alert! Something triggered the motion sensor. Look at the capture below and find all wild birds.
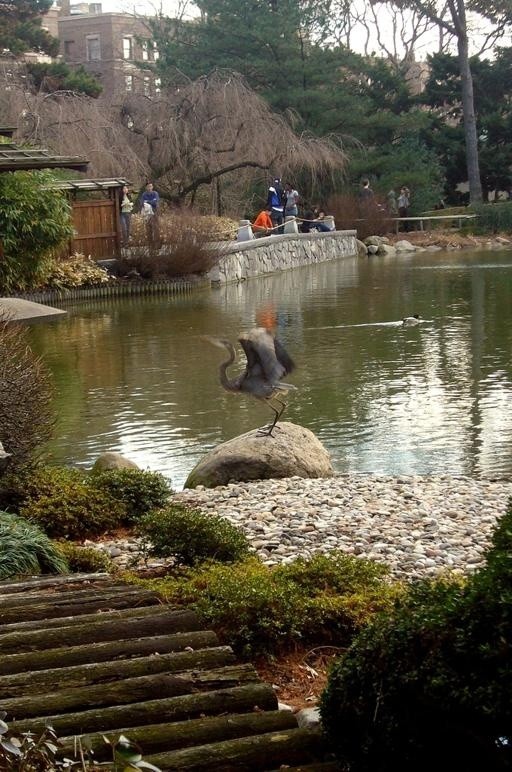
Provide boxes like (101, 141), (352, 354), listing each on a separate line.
(206, 327), (299, 438)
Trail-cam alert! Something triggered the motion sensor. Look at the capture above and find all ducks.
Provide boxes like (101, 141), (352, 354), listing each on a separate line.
(401, 315), (423, 323)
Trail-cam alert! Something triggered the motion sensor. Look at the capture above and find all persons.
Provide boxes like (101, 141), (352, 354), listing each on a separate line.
(281, 182), (300, 217)
(299, 202), (336, 233)
(267, 176), (286, 235)
(139, 182), (160, 242)
(395, 186), (411, 233)
(119, 185), (135, 249)
(253, 206), (274, 239)
(356, 178), (373, 203)
